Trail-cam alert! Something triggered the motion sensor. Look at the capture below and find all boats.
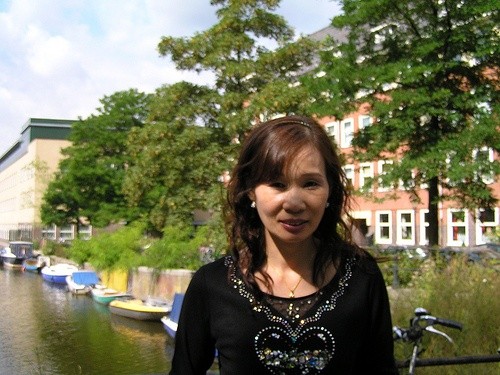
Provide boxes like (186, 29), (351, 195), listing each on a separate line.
(65, 272), (106, 295)
(41, 263), (79, 284)
(0, 241), (50, 274)
(92, 283), (136, 306)
(108, 299), (172, 322)
(160, 292), (219, 363)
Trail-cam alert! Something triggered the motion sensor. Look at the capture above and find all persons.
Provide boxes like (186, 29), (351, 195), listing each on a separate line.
(169, 114), (396, 375)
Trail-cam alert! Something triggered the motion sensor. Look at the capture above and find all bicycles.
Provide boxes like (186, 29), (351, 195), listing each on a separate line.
(391, 306), (500, 375)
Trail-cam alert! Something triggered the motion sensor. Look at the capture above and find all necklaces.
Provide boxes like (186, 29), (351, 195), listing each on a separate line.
(266, 258), (314, 299)
